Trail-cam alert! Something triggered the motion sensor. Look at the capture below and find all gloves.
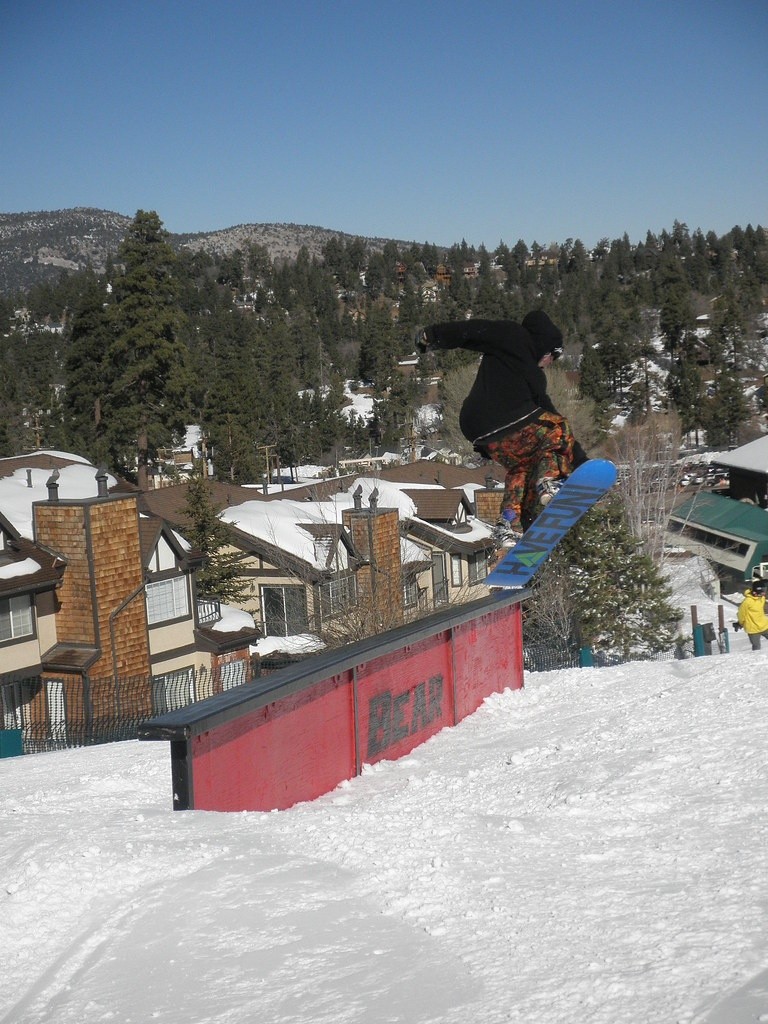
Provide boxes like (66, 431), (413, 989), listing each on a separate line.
(413, 327), (435, 355)
(732, 621), (742, 632)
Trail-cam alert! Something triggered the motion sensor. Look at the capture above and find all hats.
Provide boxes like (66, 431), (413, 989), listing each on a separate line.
(551, 340), (562, 362)
(752, 581), (766, 593)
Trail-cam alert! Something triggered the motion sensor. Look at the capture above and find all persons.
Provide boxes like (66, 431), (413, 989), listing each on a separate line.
(416, 310), (591, 550)
(737, 581), (768, 650)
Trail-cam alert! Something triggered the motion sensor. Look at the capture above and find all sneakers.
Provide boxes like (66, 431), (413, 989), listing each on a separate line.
(536, 478), (566, 506)
(497, 528), (523, 548)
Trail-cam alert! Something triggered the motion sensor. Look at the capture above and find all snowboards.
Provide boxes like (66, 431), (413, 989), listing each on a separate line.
(482, 457), (619, 586)
